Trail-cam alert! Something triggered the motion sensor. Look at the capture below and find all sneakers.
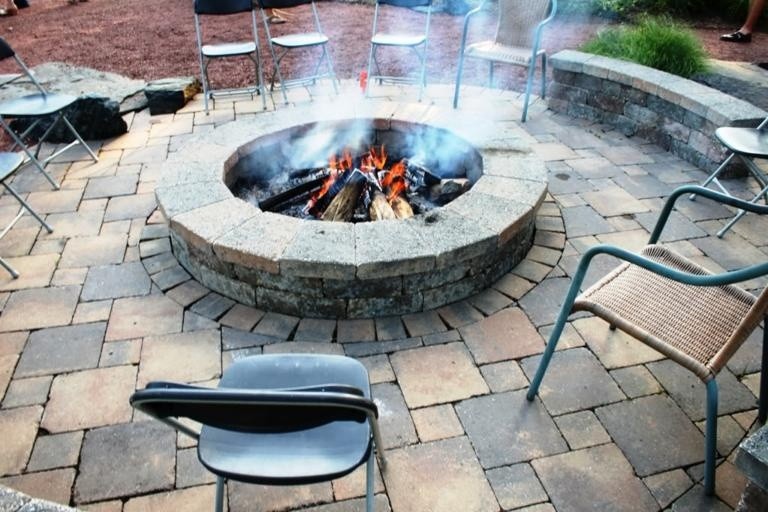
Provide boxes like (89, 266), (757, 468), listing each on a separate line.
(0, 4), (19, 16)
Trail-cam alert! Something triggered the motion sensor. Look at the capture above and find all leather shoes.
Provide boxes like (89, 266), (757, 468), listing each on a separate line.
(720, 32), (752, 44)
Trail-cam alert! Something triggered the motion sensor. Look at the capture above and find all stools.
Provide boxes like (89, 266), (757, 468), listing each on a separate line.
(547, 49), (767, 179)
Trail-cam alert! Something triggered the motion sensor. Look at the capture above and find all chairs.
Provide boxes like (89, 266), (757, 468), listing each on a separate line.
(528, 184), (768, 497)
(0, 35), (98, 191)
(1, 152), (53, 280)
(258, 1), (340, 106)
(364, 0), (433, 103)
(690, 115), (767, 237)
(453, 1), (558, 122)
(194, 1), (267, 115)
(131, 354), (386, 512)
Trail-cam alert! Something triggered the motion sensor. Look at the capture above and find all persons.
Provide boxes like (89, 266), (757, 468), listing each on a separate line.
(720, 0), (764, 42)
(264, 8), (289, 24)
(0, 0), (18, 16)
